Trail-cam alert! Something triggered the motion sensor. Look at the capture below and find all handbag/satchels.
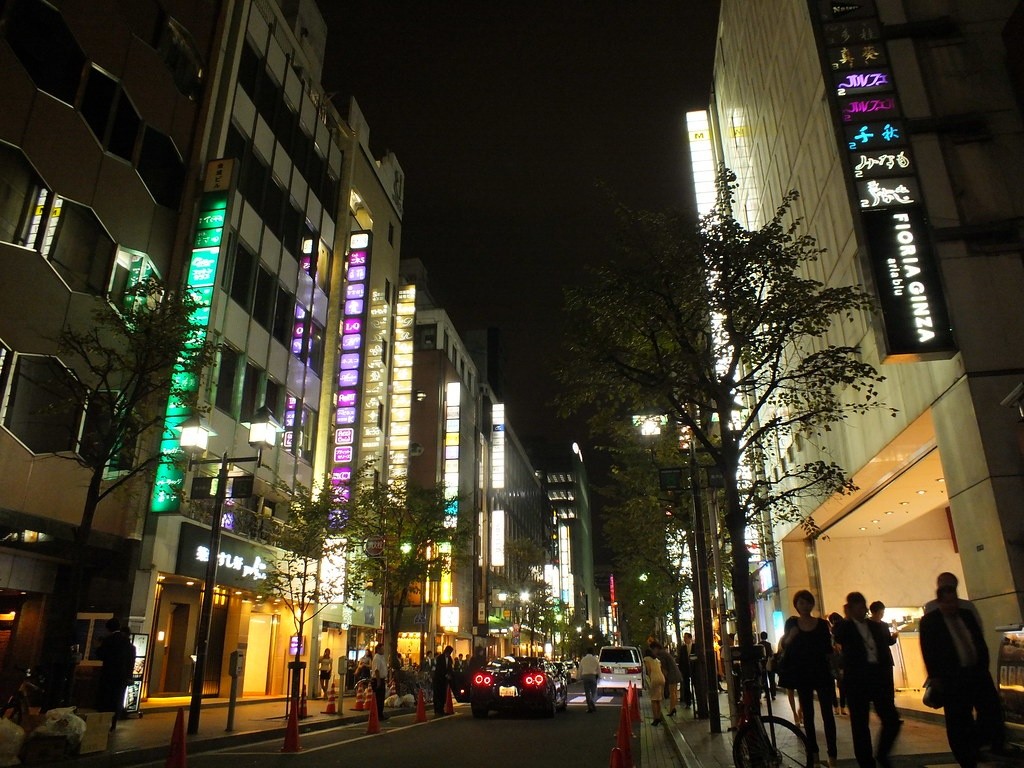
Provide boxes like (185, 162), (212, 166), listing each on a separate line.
(778, 616), (799, 689)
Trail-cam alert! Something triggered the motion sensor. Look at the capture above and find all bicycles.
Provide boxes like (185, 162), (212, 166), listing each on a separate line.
(727, 658), (814, 768)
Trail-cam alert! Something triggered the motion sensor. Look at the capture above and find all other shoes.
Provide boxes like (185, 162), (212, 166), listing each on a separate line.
(585, 707), (596, 713)
(771, 696), (775, 699)
(828, 757), (837, 768)
(835, 712), (839, 715)
(841, 712), (847, 715)
(813, 753), (820, 768)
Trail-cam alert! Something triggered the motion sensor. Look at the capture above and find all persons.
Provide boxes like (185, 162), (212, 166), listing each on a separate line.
(358, 648), (374, 679)
(318, 649), (332, 701)
(432, 645), (453, 717)
(776, 587), (899, 768)
(576, 647), (602, 713)
(423, 652), (471, 676)
(372, 643), (391, 720)
(641, 632), (697, 725)
(758, 631), (777, 699)
(917, 570), (1010, 768)
(89, 616), (137, 730)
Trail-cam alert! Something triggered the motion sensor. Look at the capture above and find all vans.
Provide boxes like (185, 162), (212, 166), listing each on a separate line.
(595, 645), (645, 697)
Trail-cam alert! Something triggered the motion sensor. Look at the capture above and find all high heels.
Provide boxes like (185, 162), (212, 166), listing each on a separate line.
(651, 717), (661, 726)
(667, 708), (676, 716)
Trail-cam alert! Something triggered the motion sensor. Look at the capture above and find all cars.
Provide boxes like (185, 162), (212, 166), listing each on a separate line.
(552, 659), (580, 684)
(469, 654), (568, 721)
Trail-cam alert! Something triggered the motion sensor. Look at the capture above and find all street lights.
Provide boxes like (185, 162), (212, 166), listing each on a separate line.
(496, 590), (530, 656)
(174, 406), (287, 737)
(418, 567), (436, 673)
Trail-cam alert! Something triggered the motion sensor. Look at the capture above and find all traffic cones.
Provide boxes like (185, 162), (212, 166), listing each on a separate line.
(320, 682), (337, 714)
(280, 696), (303, 753)
(607, 683), (641, 768)
(163, 706), (188, 768)
(364, 682), (373, 710)
(297, 684), (308, 719)
(349, 681), (365, 711)
(626, 680), (634, 705)
(363, 692), (381, 735)
(444, 687), (455, 715)
(412, 689), (427, 724)
